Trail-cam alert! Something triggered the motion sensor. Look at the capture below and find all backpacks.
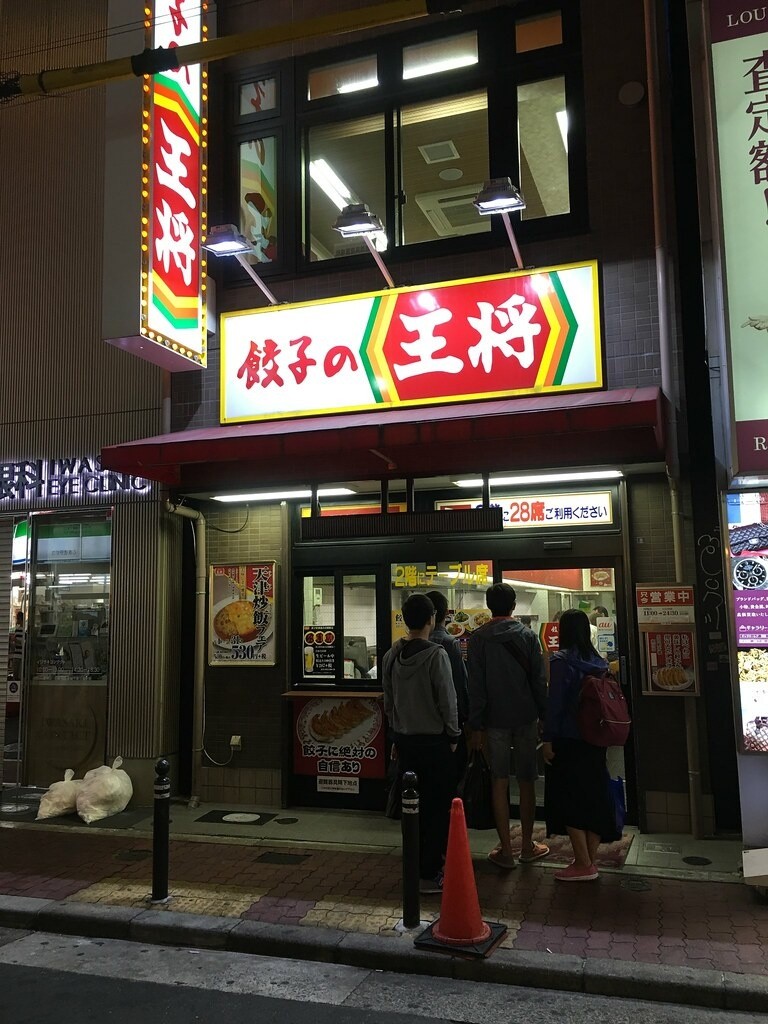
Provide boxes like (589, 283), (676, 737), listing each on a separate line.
(573, 671), (630, 748)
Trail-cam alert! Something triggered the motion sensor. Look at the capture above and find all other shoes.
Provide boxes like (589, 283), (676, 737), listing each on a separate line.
(418, 870), (444, 893)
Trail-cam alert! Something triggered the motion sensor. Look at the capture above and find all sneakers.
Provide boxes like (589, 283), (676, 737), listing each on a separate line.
(553, 863), (600, 881)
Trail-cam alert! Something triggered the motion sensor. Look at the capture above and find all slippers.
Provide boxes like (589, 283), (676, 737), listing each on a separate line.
(488, 848), (517, 868)
(519, 842), (550, 862)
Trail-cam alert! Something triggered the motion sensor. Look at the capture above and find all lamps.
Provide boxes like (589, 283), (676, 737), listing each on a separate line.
(332, 204), (396, 288)
(472, 177), (528, 271)
(307, 153), (362, 213)
(201, 227), (280, 304)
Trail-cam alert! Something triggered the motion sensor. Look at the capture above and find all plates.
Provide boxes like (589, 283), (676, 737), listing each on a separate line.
(592, 571), (609, 581)
(651, 670), (695, 691)
(296, 695), (382, 759)
(210, 595), (274, 649)
(446, 612), (491, 637)
(304, 630), (335, 645)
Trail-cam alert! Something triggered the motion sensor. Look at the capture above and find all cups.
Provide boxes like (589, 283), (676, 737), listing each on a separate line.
(304, 646), (315, 672)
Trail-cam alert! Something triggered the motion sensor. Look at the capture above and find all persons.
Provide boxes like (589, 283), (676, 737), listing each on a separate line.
(426, 583), (634, 895)
(381, 593), (461, 894)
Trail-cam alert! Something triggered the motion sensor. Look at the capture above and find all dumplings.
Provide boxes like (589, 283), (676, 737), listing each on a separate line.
(308, 698), (375, 743)
(658, 667), (688, 686)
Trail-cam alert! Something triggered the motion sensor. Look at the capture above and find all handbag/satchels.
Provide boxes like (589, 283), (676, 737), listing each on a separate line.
(386, 769), (404, 821)
(464, 746), (510, 830)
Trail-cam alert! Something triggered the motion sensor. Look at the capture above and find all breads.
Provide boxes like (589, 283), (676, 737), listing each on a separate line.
(212, 600), (268, 643)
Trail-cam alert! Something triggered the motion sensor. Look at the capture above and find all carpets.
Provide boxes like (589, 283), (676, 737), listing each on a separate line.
(492, 823), (635, 870)
